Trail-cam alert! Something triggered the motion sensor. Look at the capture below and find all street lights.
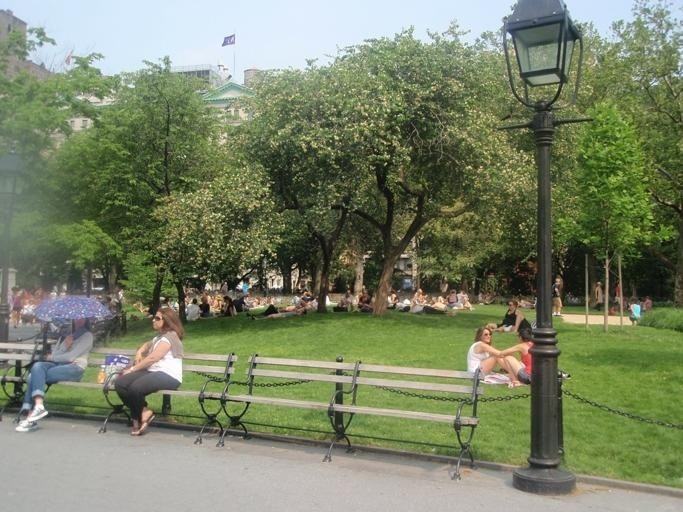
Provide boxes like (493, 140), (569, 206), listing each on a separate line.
(500, 0), (589, 496)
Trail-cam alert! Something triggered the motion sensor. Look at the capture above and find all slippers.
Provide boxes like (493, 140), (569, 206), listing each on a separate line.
(130, 411), (156, 436)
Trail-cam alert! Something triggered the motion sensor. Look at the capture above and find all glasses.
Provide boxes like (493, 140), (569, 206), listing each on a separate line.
(152, 315), (165, 321)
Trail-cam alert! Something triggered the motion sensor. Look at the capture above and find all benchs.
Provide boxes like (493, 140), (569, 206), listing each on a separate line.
(0, 340), (238, 445)
(211, 351), (482, 482)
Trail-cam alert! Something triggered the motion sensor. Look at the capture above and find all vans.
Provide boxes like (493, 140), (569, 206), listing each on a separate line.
(397, 275), (411, 292)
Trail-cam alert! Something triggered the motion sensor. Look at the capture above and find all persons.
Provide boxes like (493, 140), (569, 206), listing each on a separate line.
(93, 281), (249, 321)
(594, 280), (652, 326)
(553, 275), (563, 315)
(487, 300), (524, 332)
(116, 307), (183, 435)
(387, 289), (472, 311)
(10, 284), (60, 327)
(479, 289), (536, 308)
(466, 327), (510, 380)
(249, 289), (319, 319)
(338, 287), (374, 313)
(15, 318), (95, 433)
(500, 327), (534, 387)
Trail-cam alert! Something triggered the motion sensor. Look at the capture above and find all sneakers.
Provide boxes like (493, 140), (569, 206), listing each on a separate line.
(15, 420), (38, 433)
(26, 405), (48, 422)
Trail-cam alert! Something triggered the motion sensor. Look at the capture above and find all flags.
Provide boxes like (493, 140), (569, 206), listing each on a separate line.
(221, 34), (234, 47)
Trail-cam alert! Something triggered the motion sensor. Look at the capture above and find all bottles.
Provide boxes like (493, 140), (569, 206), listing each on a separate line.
(96, 364), (112, 385)
